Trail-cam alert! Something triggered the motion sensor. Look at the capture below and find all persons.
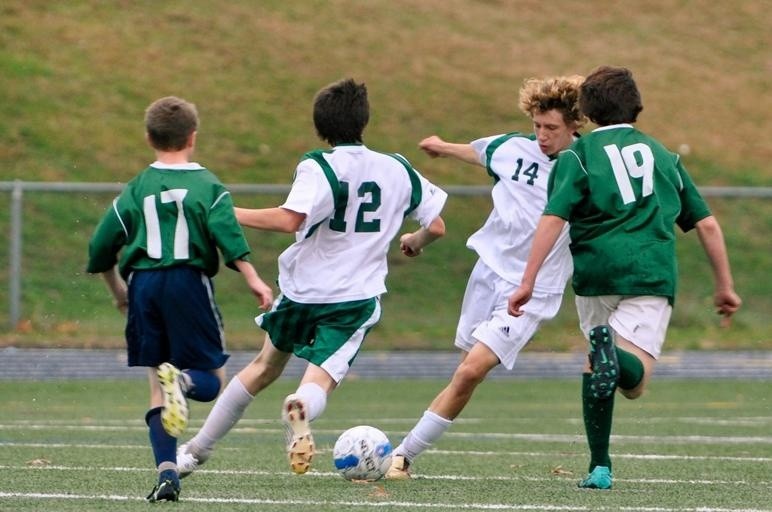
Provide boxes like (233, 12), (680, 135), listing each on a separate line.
(386, 74), (591, 479)
(85, 94), (275, 505)
(177, 76), (451, 475)
(509, 64), (743, 488)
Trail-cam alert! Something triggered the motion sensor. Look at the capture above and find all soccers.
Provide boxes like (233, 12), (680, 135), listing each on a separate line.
(332, 426), (392, 481)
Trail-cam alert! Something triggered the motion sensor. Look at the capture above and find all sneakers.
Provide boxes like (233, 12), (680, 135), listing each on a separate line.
(383, 451), (413, 481)
(586, 324), (622, 403)
(155, 361), (191, 439)
(146, 477), (182, 504)
(174, 441), (200, 481)
(281, 393), (316, 476)
(577, 464), (614, 489)
